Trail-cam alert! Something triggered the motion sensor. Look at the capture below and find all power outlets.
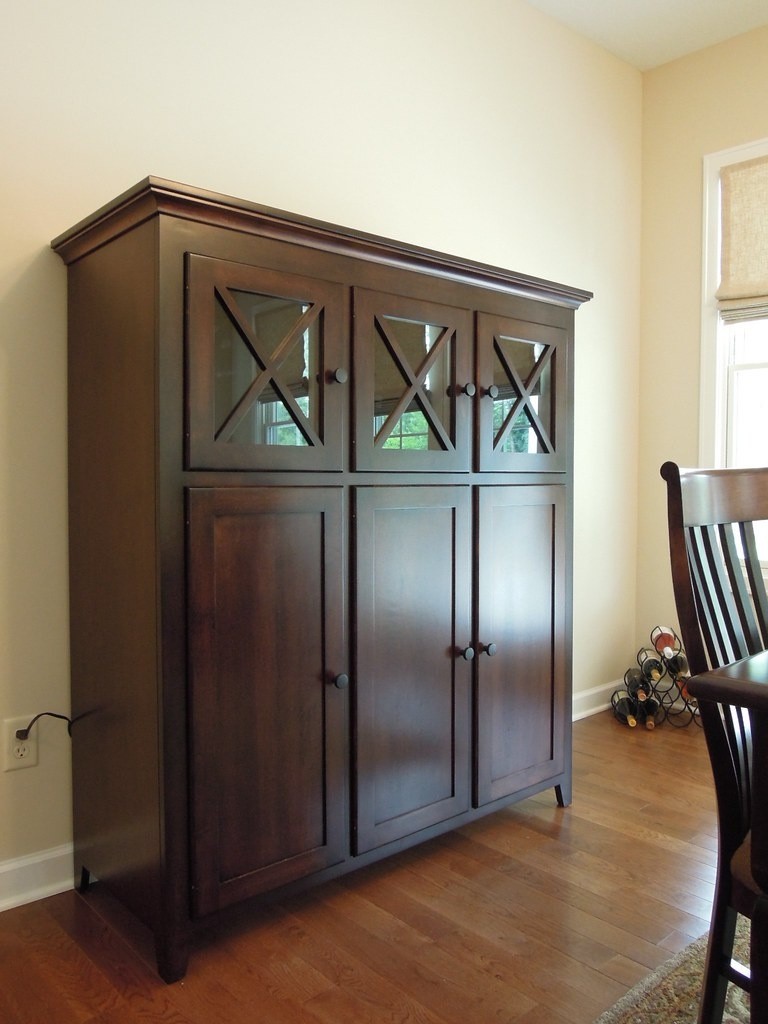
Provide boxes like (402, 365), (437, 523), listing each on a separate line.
(3, 716), (39, 770)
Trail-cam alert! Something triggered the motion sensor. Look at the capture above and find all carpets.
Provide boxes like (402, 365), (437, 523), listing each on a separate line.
(592, 912), (753, 1023)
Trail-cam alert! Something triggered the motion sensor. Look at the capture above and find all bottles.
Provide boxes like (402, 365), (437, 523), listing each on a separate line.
(639, 648), (663, 682)
(665, 648), (688, 682)
(638, 697), (661, 730)
(651, 626), (675, 659)
(626, 668), (650, 701)
(611, 690), (639, 728)
(675, 676), (697, 707)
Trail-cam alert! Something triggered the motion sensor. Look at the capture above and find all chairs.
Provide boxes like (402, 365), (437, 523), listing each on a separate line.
(657, 460), (768, 1024)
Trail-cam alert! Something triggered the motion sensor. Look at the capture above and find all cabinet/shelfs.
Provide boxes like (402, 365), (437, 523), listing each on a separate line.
(55, 176), (594, 986)
